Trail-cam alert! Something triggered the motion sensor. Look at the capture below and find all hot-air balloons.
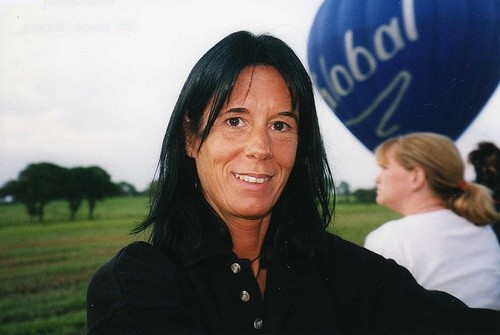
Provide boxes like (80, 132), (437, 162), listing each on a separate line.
(304, 0), (500, 154)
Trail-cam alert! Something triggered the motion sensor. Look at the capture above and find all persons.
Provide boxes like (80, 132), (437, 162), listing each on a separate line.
(85, 32), (500, 335)
(364, 132), (500, 310)
